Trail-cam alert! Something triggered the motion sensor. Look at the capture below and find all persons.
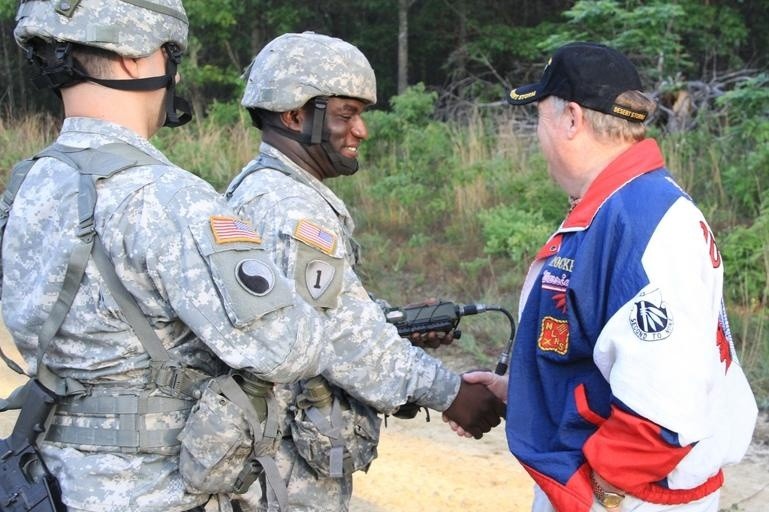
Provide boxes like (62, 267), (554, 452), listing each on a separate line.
(0, 1), (335, 512)
(442, 40), (758, 512)
(224, 30), (507, 512)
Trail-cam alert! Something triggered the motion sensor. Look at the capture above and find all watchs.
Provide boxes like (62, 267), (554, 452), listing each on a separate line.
(589, 473), (625, 509)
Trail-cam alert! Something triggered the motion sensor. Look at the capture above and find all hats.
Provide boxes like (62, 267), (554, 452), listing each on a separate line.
(508, 43), (648, 125)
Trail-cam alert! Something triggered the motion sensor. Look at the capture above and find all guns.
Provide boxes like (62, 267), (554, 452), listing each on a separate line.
(0, 378), (68, 512)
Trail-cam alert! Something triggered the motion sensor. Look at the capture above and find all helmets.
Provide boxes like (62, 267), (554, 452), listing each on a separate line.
(14, 0), (191, 58)
(241, 32), (377, 111)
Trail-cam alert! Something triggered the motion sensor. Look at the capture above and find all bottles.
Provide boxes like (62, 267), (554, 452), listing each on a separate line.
(181, 366), (284, 508)
(294, 376), (381, 472)
